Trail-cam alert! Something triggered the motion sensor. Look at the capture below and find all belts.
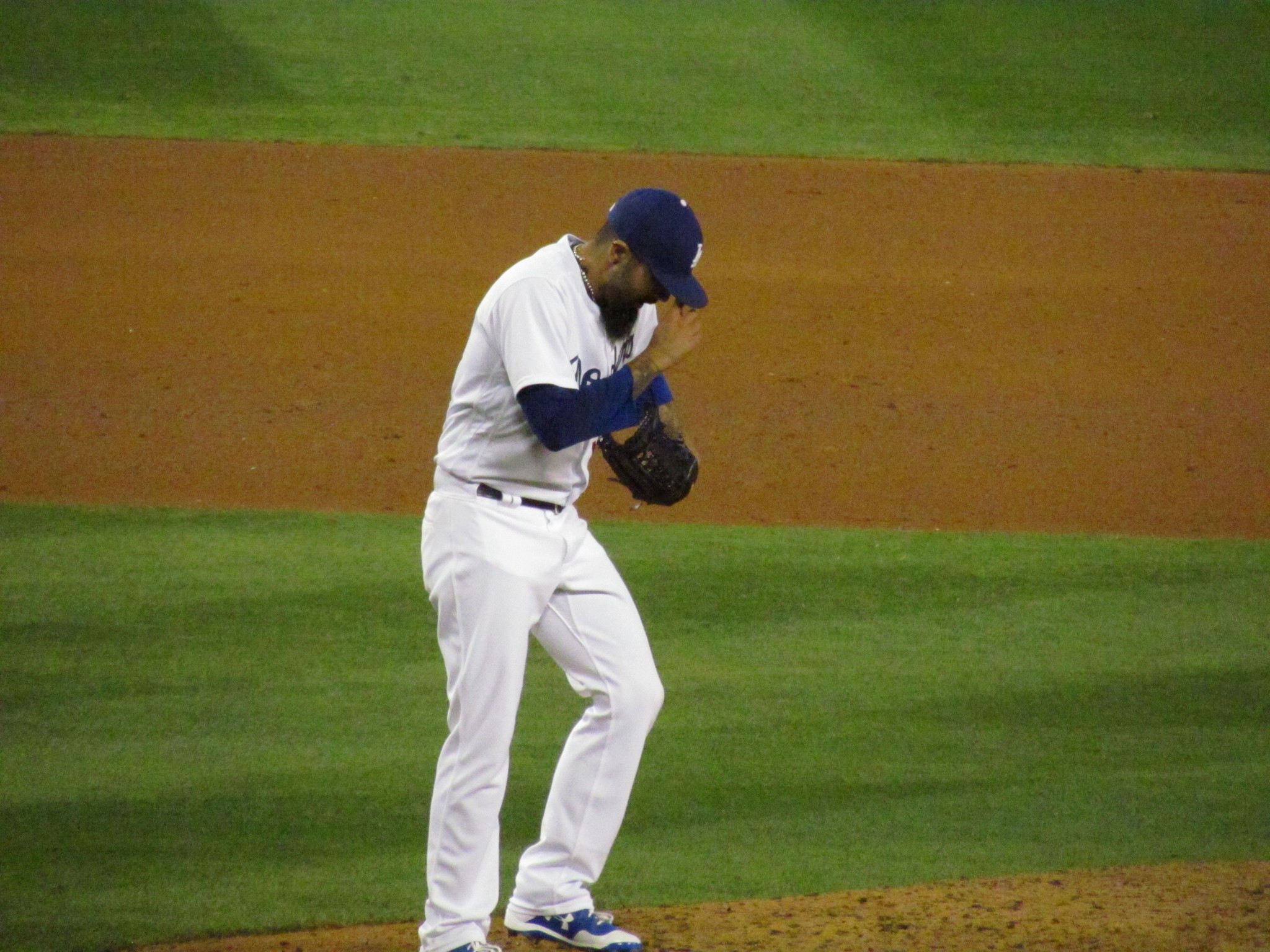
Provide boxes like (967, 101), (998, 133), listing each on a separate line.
(477, 484), (564, 514)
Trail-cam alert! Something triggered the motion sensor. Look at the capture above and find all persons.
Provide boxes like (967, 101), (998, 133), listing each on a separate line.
(418, 185), (709, 952)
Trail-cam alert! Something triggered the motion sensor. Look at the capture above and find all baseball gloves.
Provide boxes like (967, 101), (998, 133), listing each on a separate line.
(598, 403), (699, 507)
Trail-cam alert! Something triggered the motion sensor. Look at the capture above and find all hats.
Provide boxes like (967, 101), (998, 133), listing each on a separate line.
(607, 187), (708, 309)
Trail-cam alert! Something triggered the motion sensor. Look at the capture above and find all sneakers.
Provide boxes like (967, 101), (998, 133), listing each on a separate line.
(504, 908), (641, 952)
(448, 940), (502, 952)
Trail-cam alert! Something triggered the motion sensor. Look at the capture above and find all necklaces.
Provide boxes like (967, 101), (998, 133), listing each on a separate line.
(573, 243), (596, 305)
